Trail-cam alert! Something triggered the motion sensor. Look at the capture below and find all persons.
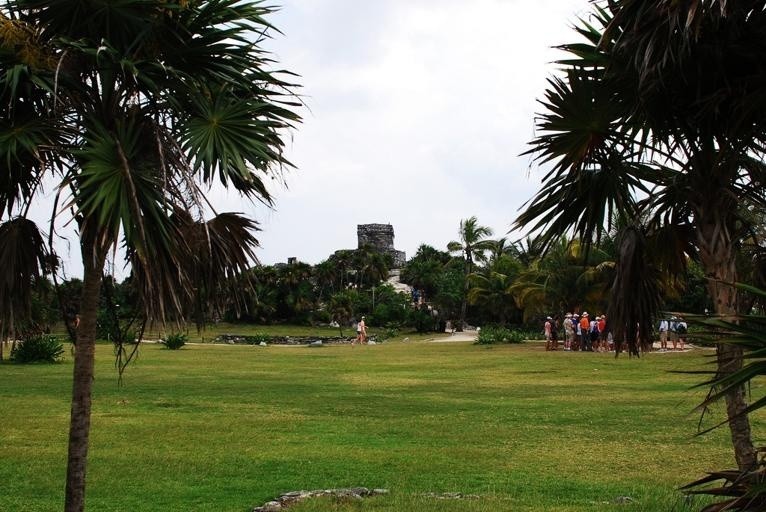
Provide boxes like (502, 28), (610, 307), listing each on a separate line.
(350, 318), (370, 347)
(75, 314), (80, 327)
(543, 310), (690, 354)
(359, 314), (369, 342)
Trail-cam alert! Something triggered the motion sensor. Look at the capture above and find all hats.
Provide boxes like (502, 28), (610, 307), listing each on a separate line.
(546, 311), (606, 320)
